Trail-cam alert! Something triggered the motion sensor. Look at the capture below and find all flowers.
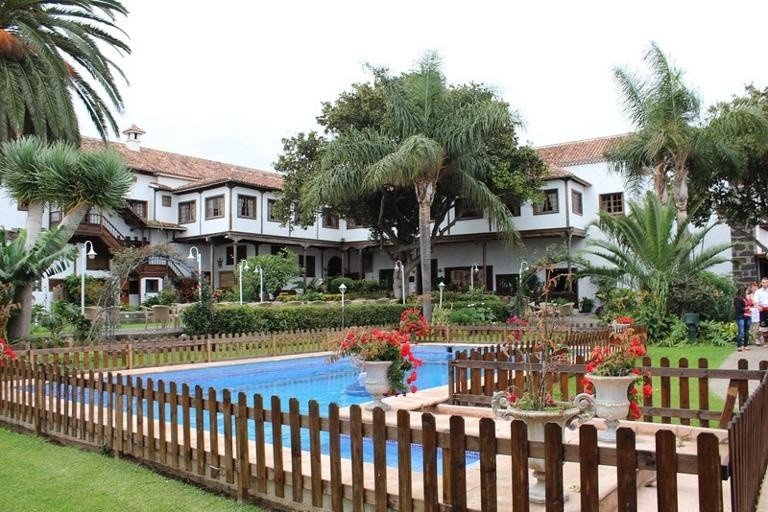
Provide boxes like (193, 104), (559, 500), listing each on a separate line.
(587, 320), (643, 375)
(336, 307), (429, 396)
(507, 315), (577, 407)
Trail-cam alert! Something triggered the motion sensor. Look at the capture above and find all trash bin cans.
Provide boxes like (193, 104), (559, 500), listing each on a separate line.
(683, 313), (700, 340)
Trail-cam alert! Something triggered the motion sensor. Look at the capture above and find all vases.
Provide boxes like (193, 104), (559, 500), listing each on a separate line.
(593, 376), (634, 442)
(495, 393), (593, 506)
(363, 361), (391, 412)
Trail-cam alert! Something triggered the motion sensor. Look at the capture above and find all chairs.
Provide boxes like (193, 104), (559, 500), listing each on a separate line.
(138, 299), (188, 331)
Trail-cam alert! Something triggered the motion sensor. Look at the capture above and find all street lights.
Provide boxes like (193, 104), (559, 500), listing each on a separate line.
(187, 245), (201, 305)
(338, 284), (346, 328)
(392, 260), (530, 307)
(80, 240), (98, 317)
(237, 258), (265, 305)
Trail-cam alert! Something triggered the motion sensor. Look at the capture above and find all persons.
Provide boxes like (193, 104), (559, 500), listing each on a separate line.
(752, 276), (768, 347)
(733, 286), (752, 351)
(745, 281), (760, 345)
